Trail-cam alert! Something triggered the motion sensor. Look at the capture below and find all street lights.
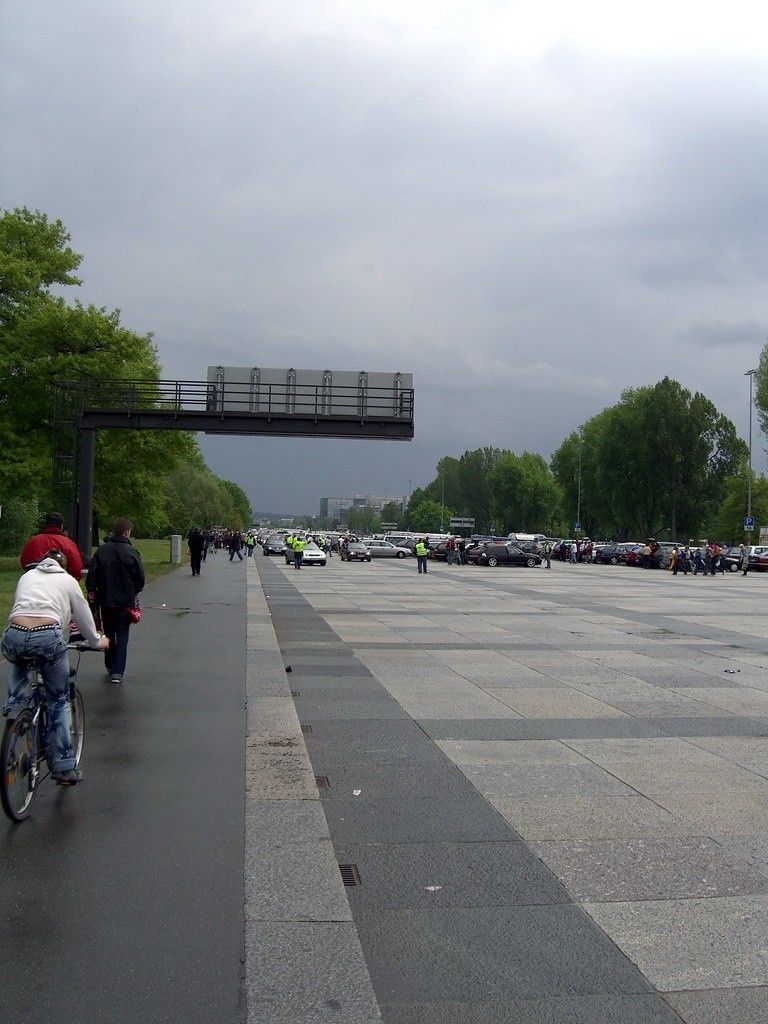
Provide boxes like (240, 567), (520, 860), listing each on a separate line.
(441, 468), (446, 534)
(744, 368), (757, 546)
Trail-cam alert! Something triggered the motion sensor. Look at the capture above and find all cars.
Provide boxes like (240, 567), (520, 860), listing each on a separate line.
(284, 541), (327, 566)
(254, 529), (768, 576)
(468, 543), (542, 567)
(340, 542), (371, 562)
(361, 539), (413, 559)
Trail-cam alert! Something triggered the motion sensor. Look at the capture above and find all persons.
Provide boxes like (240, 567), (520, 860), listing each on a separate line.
(103, 532), (113, 544)
(614, 544), (639, 552)
(668, 542), (749, 576)
(543, 540), (552, 569)
(86, 518), (145, 684)
(20, 512), (83, 582)
(285, 531), (333, 570)
(188, 528), (205, 576)
(532, 538), (544, 556)
(458, 538), (466, 565)
(202, 530), (257, 562)
(413, 538), (437, 574)
(335, 535), (380, 556)
(559, 540), (594, 564)
(522, 542), (526, 553)
(445, 536), (455, 565)
(1, 552), (109, 782)
(423, 537), (430, 546)
(641, 542), (656, 569)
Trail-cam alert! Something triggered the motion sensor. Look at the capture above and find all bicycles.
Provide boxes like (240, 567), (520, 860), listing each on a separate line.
(0, 644), (107, 823)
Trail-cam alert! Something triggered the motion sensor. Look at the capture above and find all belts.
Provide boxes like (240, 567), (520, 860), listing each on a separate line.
(10, 623), (61, 632)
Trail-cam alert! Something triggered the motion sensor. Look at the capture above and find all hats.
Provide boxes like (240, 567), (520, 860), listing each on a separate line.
(43, 550), (67, 571)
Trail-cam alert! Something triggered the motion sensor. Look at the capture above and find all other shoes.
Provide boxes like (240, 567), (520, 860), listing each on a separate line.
(110, 673), (123, 683)
(51, 768), (84, 782)
(4, 708), (24, 736)
